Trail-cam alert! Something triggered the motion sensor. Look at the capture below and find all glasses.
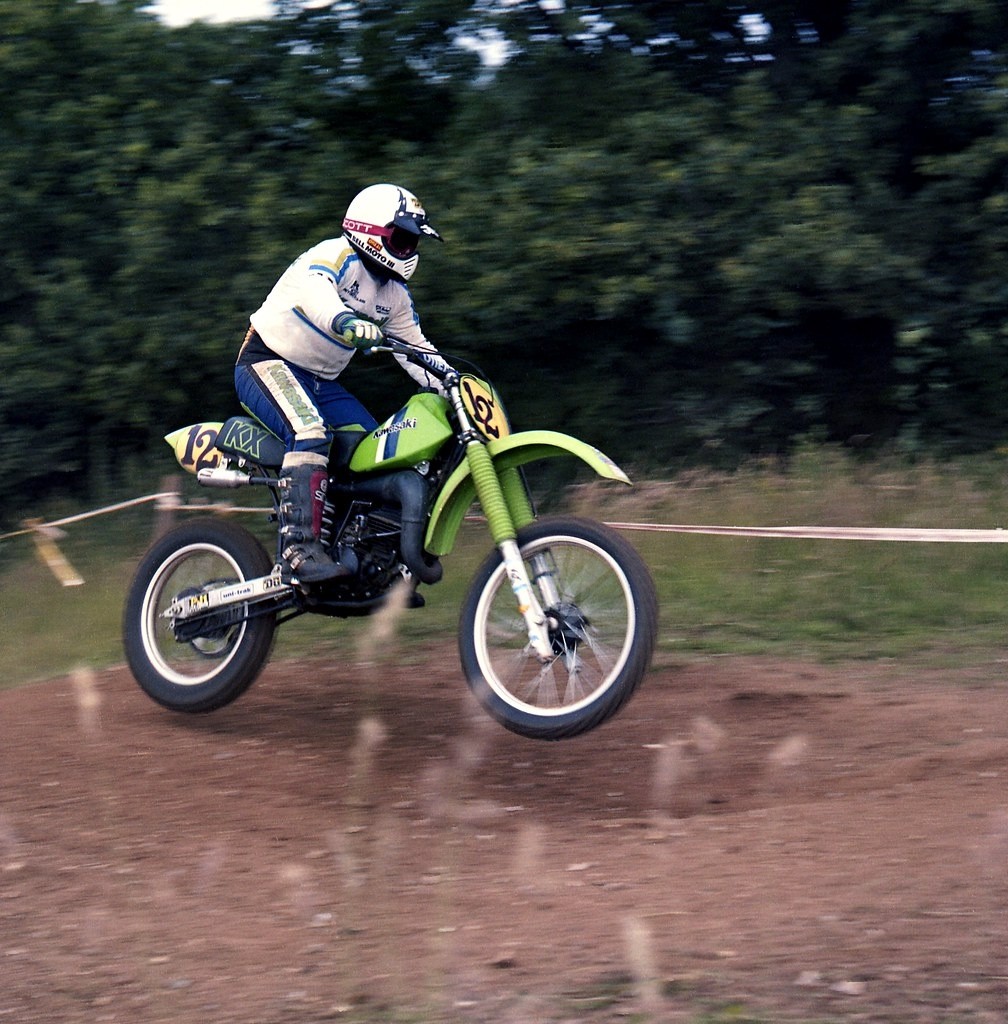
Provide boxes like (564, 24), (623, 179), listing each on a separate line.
(342, 218), (420, 256)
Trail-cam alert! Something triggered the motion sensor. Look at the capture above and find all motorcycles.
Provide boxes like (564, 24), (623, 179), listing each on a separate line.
(121, 327), (662, 741)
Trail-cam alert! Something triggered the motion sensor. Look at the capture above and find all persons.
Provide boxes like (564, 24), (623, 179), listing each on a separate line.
(236, 184), (461, 582)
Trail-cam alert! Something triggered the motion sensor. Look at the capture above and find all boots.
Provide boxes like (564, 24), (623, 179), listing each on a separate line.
(275, 451), (346, 583)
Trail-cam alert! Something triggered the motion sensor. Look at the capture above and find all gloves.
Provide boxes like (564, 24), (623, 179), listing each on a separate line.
(331, 311), (384, 350)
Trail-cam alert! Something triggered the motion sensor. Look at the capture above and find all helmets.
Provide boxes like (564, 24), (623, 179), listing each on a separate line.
(342, 184), (444, 281)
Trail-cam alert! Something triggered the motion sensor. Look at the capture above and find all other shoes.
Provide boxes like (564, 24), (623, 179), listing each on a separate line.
(399, 592), (425, 608)
